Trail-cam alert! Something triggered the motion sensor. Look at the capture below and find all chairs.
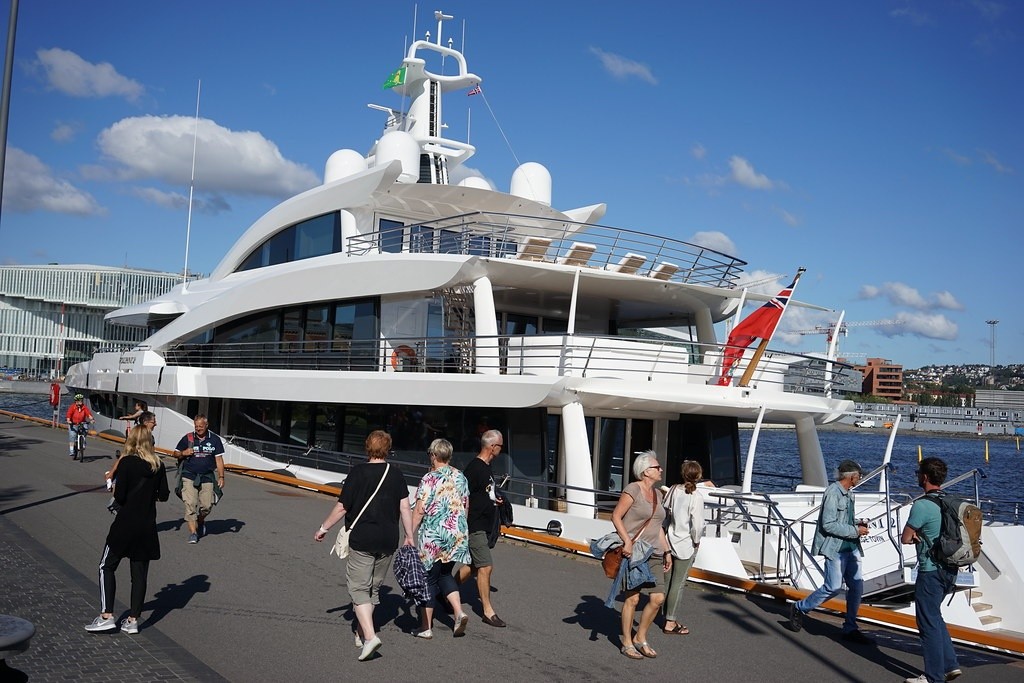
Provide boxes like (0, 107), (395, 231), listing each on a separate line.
(648, 261), (679, 280)
(601, 253), (647, 274)
(506, 237), (552, 262)
(556, 242), (596, 266)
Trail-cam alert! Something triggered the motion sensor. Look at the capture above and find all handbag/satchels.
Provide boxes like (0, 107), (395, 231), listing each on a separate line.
(601, 545), (623, 579)
(330, 526), (349, 560)
(662, 485), (678, 533)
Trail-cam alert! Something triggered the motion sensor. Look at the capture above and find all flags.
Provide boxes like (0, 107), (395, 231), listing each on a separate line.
(382, 67), (406, 89)
(716, 276), (796, 387)
(468, 86), (481, 95)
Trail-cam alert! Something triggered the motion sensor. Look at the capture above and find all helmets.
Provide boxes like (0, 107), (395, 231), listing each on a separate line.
(73, 394), (84, 401)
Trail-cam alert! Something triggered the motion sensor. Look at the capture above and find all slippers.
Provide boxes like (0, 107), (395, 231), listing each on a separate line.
(621, 641), (657, 659)
(664, 622), (689, 634)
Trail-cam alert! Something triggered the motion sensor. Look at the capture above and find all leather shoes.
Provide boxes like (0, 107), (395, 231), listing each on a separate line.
(481, 612), (506, 627)
(436, 591), (454, 614)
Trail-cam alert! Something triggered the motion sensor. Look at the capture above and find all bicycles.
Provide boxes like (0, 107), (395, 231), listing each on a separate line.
(66, 421), (91, 462)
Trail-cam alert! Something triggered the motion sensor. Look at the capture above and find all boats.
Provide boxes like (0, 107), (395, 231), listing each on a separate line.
(63, 2), (1024, 662)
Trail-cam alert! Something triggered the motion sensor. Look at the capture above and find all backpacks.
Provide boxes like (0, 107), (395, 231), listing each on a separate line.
(913, 494), (982, 568)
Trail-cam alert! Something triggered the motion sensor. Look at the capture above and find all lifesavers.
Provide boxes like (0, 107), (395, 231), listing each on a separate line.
(391, 345), (417, 369)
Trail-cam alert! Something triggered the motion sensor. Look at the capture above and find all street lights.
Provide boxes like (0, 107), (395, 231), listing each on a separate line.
(985, 320), (999, 365)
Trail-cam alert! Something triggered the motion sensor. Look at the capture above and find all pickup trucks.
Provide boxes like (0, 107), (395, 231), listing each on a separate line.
(883, 422), (894, 429)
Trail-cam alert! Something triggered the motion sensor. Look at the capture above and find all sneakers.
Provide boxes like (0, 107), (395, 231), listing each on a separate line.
(905, 669), (961, 683)
(196, 524), (207, 535)
(85, 616), (139, 634)
(790, 603), (803, 632)
(840, 629), (874, 644)
(188, 533), (198, 544)
(355, 635), (382, 661)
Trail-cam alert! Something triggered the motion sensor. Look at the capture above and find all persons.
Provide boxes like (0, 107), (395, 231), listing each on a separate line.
(84, 402), (170, 635)
(900, 457), (963, 683)
(662, 460), (705, 636)
(173, 413), (225, 544)
(790, 459), (869, 642)
(314, 431), (416, 661)
(66, 395), (95, 456)
(612, 450), (673, 660)
(403, 439), (470, 640)
(454, 430), (513, 627)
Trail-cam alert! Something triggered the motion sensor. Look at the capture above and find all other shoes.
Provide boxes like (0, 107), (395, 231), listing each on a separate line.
(83, 443), (86, 450)
(69, 449), (75, 456)
(454, 613), (468, 637)
(411, 628), (433, 639)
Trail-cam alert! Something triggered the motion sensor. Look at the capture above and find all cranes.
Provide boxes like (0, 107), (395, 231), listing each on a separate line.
(787, 320), (906, 360)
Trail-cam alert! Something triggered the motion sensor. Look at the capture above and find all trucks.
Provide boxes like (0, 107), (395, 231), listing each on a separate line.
(855, 420), (875, 428)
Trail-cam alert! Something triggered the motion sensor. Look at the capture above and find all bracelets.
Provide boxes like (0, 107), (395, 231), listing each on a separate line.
(319, 524), (329, 534)
(219, 476), (224, 478)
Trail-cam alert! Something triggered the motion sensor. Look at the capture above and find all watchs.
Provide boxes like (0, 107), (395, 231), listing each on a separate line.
(663, 550), (671, 556)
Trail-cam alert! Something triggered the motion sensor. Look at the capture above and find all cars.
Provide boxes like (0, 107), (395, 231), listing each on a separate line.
(0, 367), (49, 381)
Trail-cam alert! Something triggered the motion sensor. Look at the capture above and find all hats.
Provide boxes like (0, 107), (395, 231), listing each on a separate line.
(838, 459), (868, 476)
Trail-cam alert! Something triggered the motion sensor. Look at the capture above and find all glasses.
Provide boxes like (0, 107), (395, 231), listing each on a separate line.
(650, 464), (661, 470)
(426, 452), (437, 457)
(491, 443), (505, 449)
(853, 475), (862, 480)
(915, 469), (925, 477)
(146, 421), (156, 424)
